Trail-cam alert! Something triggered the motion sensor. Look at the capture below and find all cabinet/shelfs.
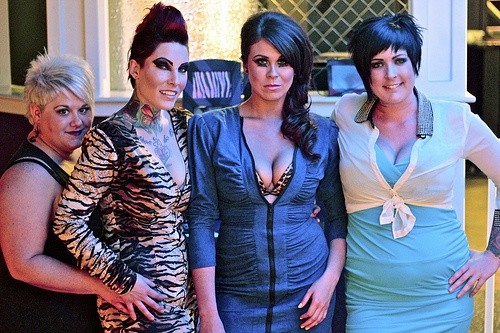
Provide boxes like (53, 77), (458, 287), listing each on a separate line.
(468, 39), (500, 177)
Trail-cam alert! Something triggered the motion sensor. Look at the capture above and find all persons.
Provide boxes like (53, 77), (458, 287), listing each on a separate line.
(0, 45), (131, 333)
(52, 1), (320, 333)
(186, 10), (348, 333)
(331, 10), (500, 333)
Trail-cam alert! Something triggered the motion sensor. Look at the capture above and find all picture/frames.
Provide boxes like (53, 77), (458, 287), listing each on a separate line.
(181, 59), (243, 110)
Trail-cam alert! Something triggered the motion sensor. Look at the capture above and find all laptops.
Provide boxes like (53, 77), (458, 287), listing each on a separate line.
(326, 58), (368, 98)
